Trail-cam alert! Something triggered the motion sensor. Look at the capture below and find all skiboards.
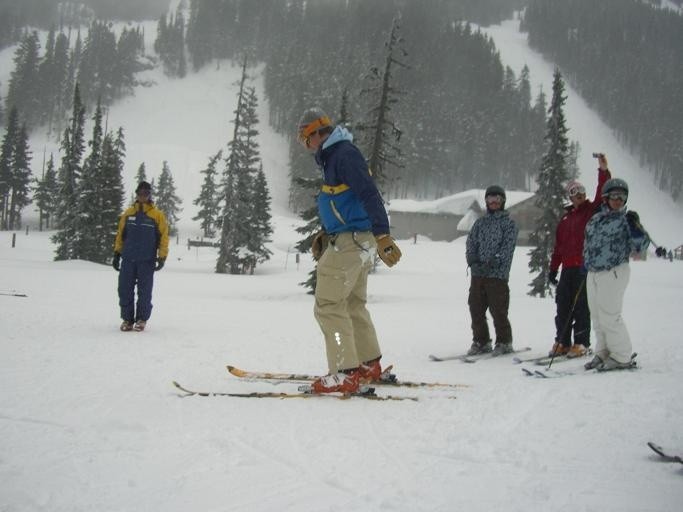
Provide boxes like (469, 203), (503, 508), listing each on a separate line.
(647, 441), (683, 462)
(174, 366), (474, 402)
(428, 348), (637, 377)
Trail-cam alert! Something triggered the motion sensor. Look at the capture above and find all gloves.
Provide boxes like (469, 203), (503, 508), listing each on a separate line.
(311, 230), (329, 261)
(625, 209), (644, 239)
(548, 271), (559, 284)
(375, 233), (402, 268)
(154, 257), (165, 272)
(112, 253), (121, 272)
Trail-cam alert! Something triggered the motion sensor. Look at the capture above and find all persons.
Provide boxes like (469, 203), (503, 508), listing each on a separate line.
(582, 177), (652, 372)
(295, 107), (402, 392)
(112, 182), (169, 333)
(548, 152), (611, 359)
(465, 184), (517, 357)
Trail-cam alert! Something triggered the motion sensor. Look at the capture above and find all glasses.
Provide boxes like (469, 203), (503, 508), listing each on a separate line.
(600, 193), (628, 202)
(569, 186), (586, 196)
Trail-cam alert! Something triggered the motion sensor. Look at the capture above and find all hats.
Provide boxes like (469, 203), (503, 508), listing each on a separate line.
(601, 177), (630, 193)
(135, 181), (153, 193)
(566, 179), (583, 192)
(485, 184), (505, 197)
(296, 106), (332, 144)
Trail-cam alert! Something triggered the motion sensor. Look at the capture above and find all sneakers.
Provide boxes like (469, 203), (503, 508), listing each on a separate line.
(119, 320), (133, 331)
(584, 355), (602, 370)
(491, 341), (513, 354)
(548, 340), (571, 357)
(359, 360), (382, 381)
(467, 341), (493, 354)
(566, 343), (589, 358)
(134, 319), (146, 331)
(313, 371), (360, 393)
(596, 357), (635, 371)
